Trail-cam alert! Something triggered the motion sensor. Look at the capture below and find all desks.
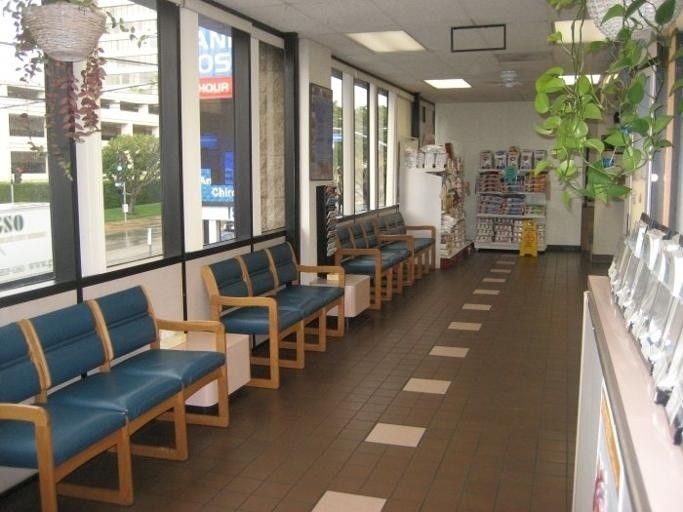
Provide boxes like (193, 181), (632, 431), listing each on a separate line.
(163, 332), (252, 410)
(310, 273), (370, 323)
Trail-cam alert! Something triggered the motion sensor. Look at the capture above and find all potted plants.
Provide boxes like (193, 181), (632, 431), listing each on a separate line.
(533, 0), (683, 209)
(0, 0), (159, 183)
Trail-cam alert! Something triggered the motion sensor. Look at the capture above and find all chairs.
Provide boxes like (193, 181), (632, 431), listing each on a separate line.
(375, 212), (435, 286)
(1, 286), (230, 512)
(201, 241), (345, 389)
(334, 218), (412, 311)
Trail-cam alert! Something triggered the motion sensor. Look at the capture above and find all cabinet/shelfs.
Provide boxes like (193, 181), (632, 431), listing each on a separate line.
(571, 275), (683, 512)
(475, 168), (549, 252)
(400, 163), (471, 269)
(317, 184), (337, 277)
